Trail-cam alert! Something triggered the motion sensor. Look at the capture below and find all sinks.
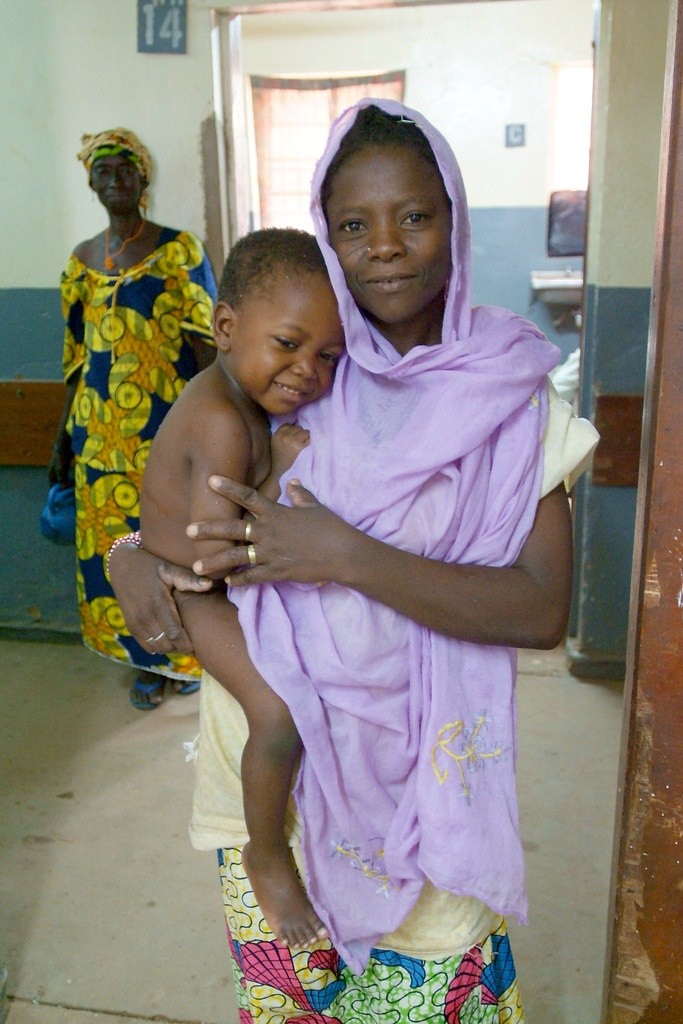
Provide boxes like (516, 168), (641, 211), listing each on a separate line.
(530, 270), (583, 305)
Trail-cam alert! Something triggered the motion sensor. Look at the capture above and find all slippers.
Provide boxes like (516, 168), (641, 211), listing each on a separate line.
(170, 678), (201, 694)
(129, 674), (165, 709)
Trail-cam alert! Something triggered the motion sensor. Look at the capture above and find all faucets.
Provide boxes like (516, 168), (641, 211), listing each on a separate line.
(563, 265), (573, 277)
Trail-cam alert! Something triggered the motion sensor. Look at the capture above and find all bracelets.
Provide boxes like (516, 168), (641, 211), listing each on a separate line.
(54, 443), (74, 459)
(106, 531), (143, 572)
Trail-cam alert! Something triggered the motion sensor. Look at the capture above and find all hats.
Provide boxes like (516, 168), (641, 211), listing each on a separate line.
(76, 127), (151, 209)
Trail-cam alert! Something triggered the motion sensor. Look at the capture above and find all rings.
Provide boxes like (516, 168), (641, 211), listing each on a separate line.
(248, 545), (256, 564)
(144, 637), (153, 642)
(245, 519), (252, 541)
(153, 632), (165, 642)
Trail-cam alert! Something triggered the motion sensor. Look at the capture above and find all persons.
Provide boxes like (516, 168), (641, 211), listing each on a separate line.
(47, 127), (221, 708)
(137, 228), (346, 954)
(108, 95), (601, 1024)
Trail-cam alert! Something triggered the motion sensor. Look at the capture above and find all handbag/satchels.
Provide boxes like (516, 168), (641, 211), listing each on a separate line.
(41, 482), (77, 546)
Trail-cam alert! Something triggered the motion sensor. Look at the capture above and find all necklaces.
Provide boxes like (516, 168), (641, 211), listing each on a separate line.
(104, 221), (146, 270)
